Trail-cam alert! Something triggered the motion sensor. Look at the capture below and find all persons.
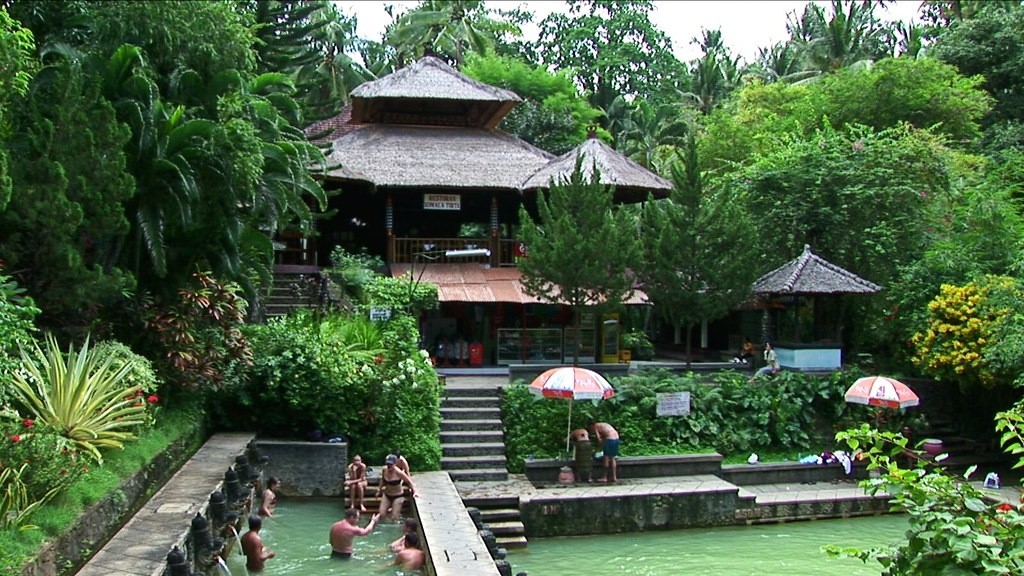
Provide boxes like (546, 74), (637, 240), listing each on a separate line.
(739, 336), (780, 377)
(238, 450), (425, 571)
(565, 421), (619, 482)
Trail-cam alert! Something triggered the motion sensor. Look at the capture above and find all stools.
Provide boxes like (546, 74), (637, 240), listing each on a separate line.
(984, 475), (1000, 489)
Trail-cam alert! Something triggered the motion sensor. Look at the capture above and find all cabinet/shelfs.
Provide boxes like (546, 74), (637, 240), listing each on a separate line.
(564, 327), (596, 363)
(496, 328), (563, 366)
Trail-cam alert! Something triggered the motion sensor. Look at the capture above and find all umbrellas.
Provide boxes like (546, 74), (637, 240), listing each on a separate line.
(844, 376), (920, 431)
(529, 368), (615, 467)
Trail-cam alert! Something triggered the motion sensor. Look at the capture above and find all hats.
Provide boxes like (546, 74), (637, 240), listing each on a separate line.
(385, 454), (397, 463)
